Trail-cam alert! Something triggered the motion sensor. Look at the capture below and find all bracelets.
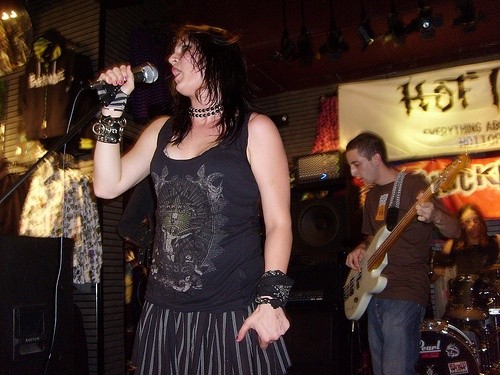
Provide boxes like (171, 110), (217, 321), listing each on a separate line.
(91, 113), (128, 144)
(253, 269), (294, 308)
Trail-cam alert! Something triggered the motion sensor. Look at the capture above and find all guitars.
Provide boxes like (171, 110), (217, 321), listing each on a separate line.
(342, 153), (469, 321)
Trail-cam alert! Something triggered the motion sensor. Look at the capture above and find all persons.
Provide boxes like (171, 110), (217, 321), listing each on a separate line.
(433, 202), (499, 288)
(345, 131), (462, 375)
(91, 24), (300, 375)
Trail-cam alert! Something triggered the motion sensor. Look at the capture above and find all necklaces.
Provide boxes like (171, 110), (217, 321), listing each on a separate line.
(187, 102), (223, 118)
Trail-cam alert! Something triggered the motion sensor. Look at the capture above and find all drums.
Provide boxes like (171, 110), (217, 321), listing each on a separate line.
(418, 321), (482, 375)
(445, 276), (490, 320)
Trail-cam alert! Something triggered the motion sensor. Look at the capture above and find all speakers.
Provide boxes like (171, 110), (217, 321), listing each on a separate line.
(0, 234), (75, 375)
(287, 177), (363, 291)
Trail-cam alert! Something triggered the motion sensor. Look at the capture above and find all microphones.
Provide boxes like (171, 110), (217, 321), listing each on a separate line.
(80, 62), (158, 91)
